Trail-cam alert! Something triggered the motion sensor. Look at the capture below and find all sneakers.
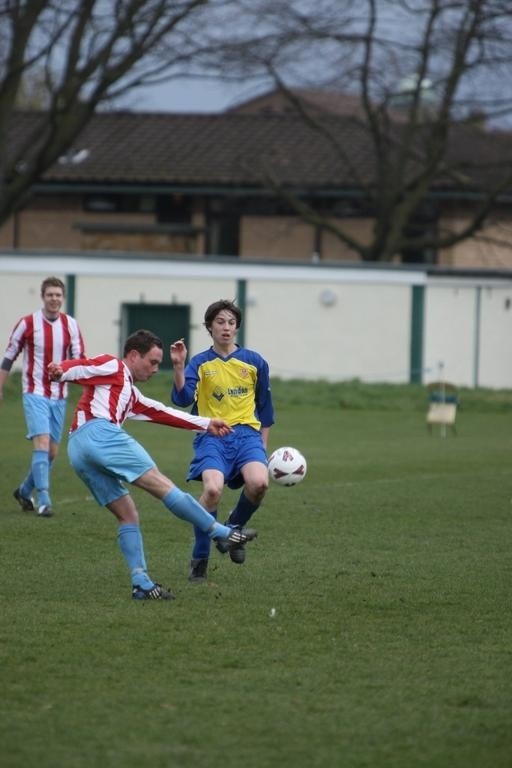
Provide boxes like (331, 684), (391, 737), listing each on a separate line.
(130, 583), (176, 601)
(189, 558), (209, 584)
(216, 526), (256, 553)
(225, 516), (248, 564)
(36, 505), (53, 519)
(13, 489), (37, 512)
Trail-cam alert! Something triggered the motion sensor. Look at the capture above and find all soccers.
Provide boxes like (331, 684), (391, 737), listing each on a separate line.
(267, 447), (306, 487)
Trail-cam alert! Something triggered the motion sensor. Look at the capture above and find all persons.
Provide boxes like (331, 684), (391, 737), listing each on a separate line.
(48, 330), (258, 603)
(0, 277), (84, 517)
(171, 299), (274, 581)
